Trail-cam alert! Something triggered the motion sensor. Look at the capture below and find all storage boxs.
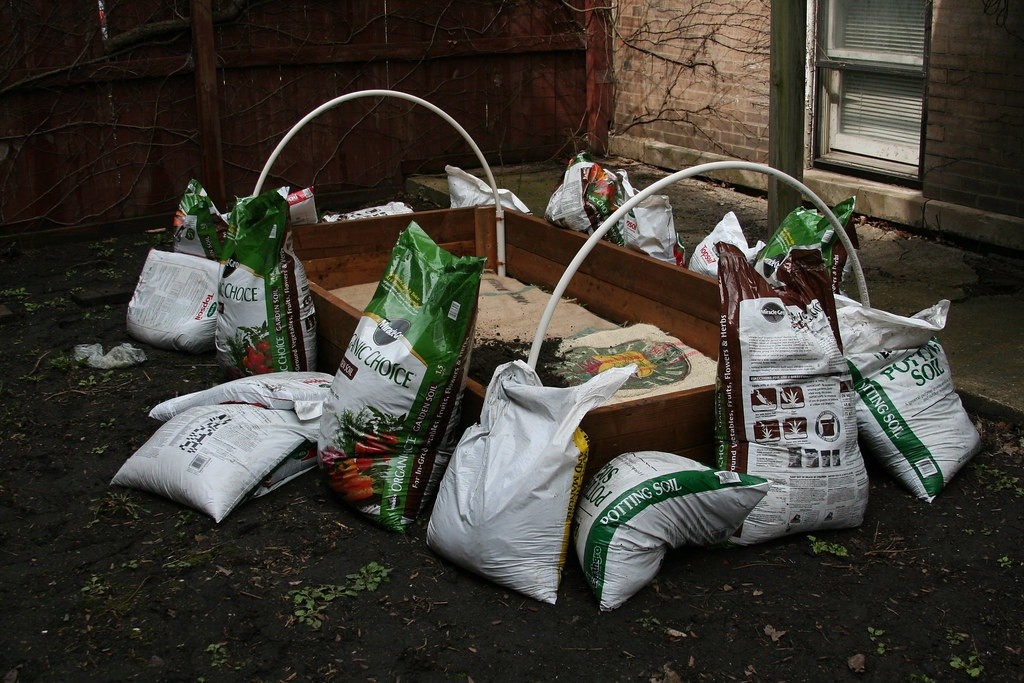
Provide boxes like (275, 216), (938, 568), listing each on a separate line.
(281, 201), (719, 470)
